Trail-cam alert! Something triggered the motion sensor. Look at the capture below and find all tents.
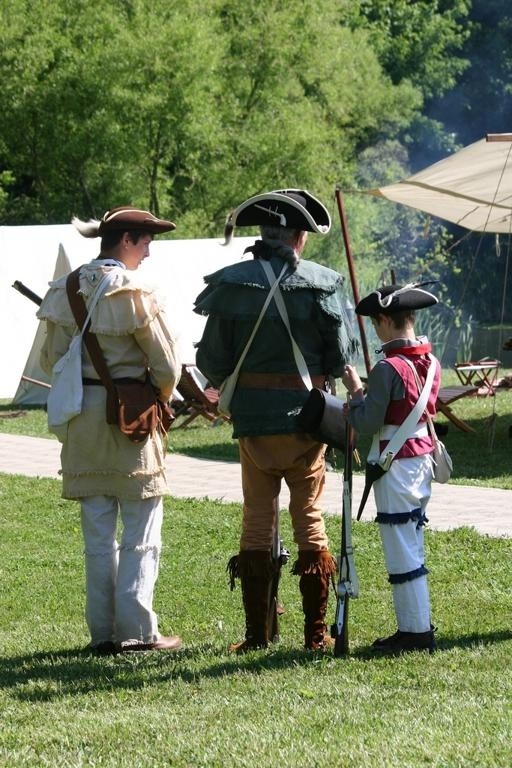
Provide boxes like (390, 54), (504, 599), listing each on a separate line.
(14, 236), (262, 405)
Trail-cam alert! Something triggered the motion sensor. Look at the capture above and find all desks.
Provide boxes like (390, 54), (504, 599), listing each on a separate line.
(454, 363), (498, 398)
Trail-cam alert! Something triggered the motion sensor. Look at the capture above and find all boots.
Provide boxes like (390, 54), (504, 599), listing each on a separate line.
(236, 550), (282, 651)
(296, 549), (333, 649)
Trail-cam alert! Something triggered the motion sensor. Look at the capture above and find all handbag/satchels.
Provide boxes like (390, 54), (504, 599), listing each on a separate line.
(214, 372), (239, 419)
(304, 386), (349, 448)
(106, 377), (174, 442)
(47, 334), (82, 425)
(432, 436), (453, 484)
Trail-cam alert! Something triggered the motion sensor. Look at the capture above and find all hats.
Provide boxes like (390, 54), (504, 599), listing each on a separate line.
(355, 285), (439, 314)
(100, 207), (176, 234)
(232, 189), (331, 235)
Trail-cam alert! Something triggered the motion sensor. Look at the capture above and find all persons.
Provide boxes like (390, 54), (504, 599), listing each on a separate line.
(36, 207), (183, 651)
(194, 190), (361, 653)
(342, 285), (441, 654)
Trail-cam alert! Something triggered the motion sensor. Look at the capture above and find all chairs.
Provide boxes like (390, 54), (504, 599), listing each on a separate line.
(359, 377), (480, 434)
(171, 376), (215, 421)
(178, 363), (233, 427)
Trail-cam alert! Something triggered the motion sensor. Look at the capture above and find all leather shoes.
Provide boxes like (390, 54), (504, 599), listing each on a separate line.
(375, 632), (439, 655)
(86, 641), (117, 654)
(120, 634), (179, 654)
